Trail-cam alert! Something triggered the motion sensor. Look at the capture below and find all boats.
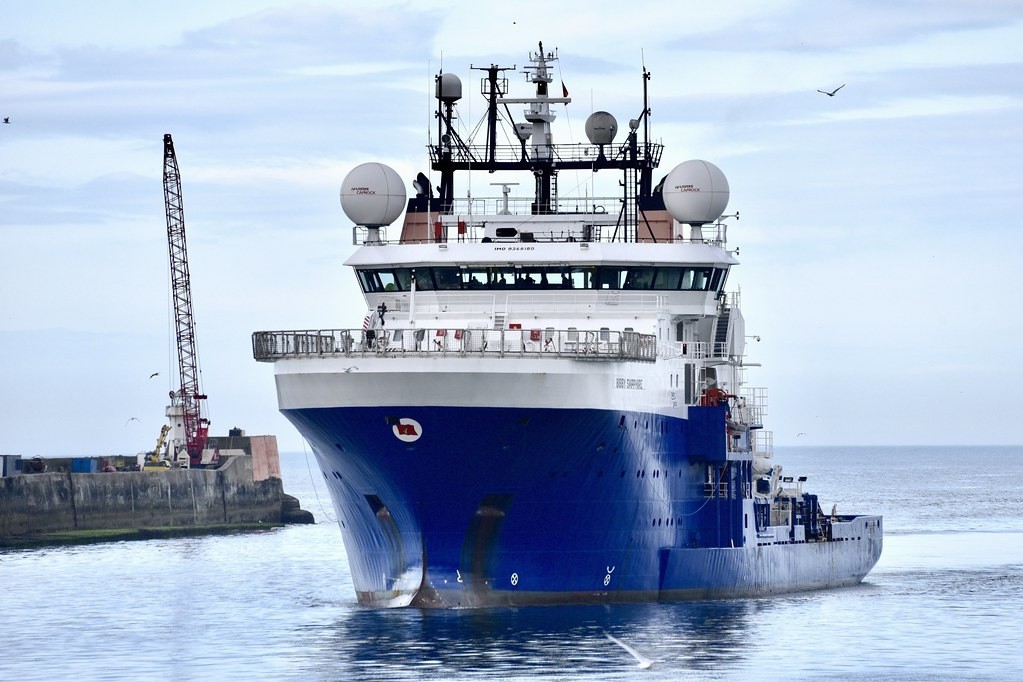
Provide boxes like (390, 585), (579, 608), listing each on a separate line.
(250, 42), (885, 610)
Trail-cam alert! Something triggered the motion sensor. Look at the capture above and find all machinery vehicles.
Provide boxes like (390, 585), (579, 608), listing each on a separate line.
(142, 135), (220, 470)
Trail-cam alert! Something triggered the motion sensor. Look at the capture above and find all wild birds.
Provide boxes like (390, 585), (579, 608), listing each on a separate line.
(3, 117), (11, 123)
(124, 417), (143, 426)
(816, 84), (846, 97)
(150, 372), (158, 380)
